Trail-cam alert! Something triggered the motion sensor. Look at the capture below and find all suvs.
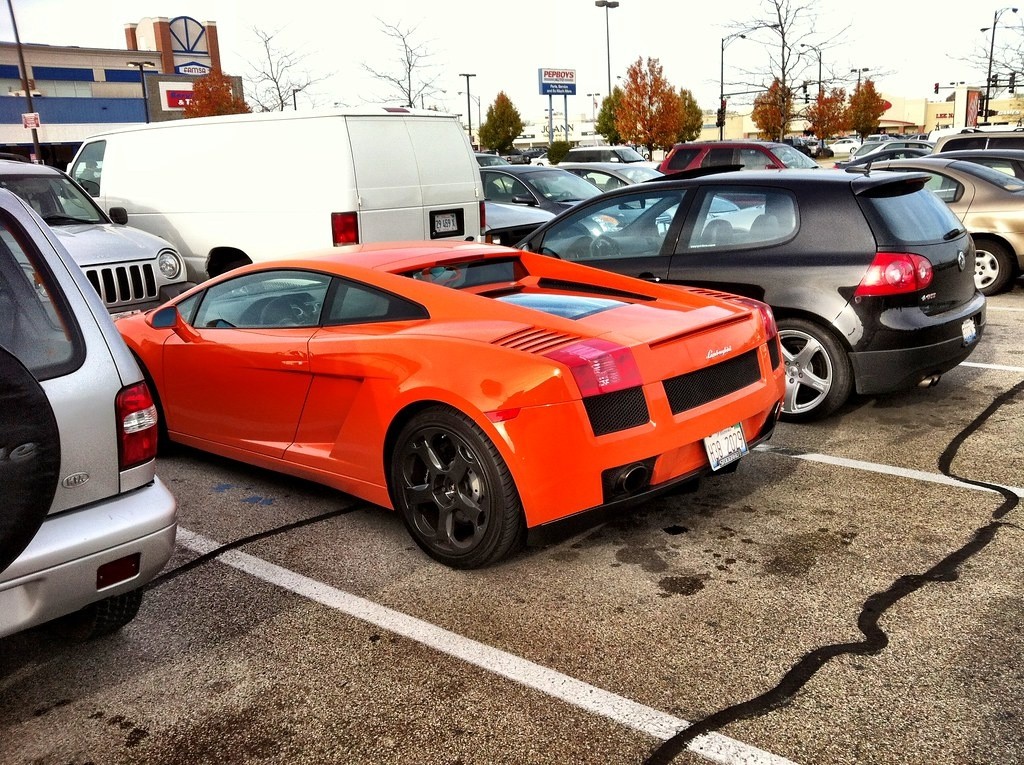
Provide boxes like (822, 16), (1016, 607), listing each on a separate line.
(1, 150), (198, 330)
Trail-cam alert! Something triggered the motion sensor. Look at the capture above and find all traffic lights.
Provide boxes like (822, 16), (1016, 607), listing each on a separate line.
(992, 74), (998, 87)
(935, 82), (939, 94)
(1009, 72), (1015, 93)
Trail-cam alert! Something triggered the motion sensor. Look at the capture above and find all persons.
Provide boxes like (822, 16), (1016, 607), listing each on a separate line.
(79, 160), (98, 179)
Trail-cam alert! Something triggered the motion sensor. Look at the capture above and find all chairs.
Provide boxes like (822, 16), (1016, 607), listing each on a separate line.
(701, 214), (780, 246)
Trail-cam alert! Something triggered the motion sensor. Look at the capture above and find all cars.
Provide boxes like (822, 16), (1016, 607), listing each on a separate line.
(449, 160), (986, 425)
(1, 185), (179, 647)
(473, 123), (1023, 299)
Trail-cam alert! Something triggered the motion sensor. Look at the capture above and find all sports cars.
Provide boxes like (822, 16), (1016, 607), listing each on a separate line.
(115, 240), (785, 573)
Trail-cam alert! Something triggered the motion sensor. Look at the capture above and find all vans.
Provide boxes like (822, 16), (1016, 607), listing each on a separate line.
(58, 106), (487, 296)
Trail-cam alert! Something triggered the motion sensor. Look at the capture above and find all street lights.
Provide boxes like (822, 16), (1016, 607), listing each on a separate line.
(587, 93), (600, 121)
(720, 33), (746, 138)
(800, 43), (822, 108)
(459, 73), (476, 134)
(292, 88), (301, 110)
(594, 0), (619, 98)
(984, 6), (1019, 122)
(851, 68), (869, 95)
(127, 61), (156, 124)
(458, 91), (481, 153)
